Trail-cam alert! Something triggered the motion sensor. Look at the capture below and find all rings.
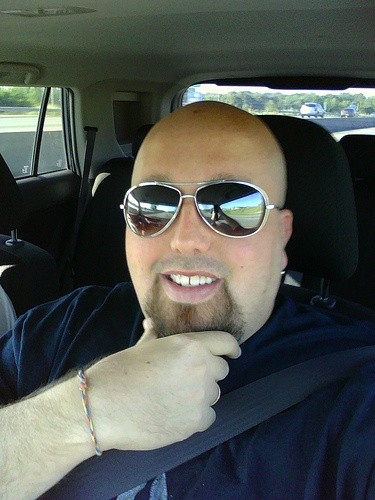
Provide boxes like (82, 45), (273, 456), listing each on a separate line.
(211, 383), (221, 406)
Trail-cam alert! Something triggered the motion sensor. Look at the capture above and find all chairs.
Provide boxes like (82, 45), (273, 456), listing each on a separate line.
(0, 115), (375, 321)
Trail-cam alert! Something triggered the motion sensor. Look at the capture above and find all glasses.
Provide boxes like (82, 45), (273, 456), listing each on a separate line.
(120, 180), (281, 238)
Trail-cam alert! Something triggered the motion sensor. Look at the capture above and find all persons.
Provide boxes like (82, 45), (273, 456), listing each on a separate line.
(0, 100), (375, 500)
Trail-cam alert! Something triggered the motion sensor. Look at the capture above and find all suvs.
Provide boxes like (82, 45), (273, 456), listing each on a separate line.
(300, 103), (325, 118)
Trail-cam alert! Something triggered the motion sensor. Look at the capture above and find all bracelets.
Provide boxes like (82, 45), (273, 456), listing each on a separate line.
(78, 370), (103, 456)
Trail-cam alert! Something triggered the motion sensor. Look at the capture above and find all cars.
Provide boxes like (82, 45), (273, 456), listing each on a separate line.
(341, 108), (358, 118)
